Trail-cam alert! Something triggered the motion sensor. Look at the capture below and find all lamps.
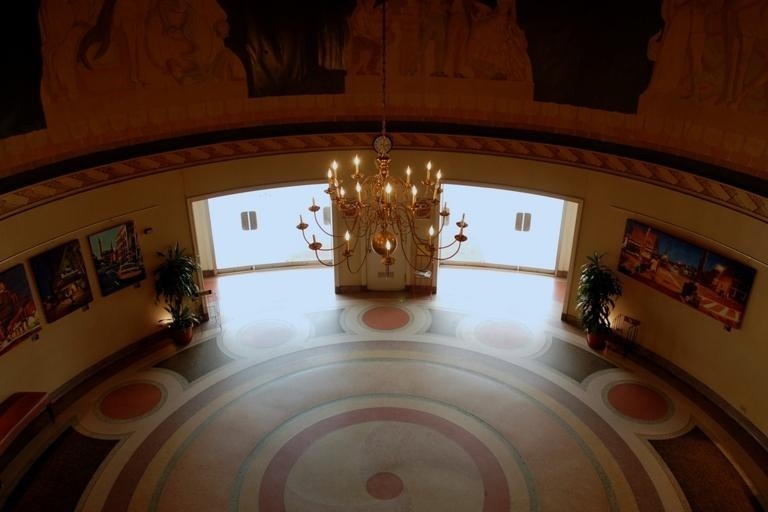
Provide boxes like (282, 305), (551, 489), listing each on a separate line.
(295, 1), (470, 281)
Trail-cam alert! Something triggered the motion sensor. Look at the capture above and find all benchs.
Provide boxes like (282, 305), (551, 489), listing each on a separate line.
(0, 390), (57, 464)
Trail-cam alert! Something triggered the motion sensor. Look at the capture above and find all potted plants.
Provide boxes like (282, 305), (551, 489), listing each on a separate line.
(147, 241), (207, 346)
(570, 248), (625, 357)
